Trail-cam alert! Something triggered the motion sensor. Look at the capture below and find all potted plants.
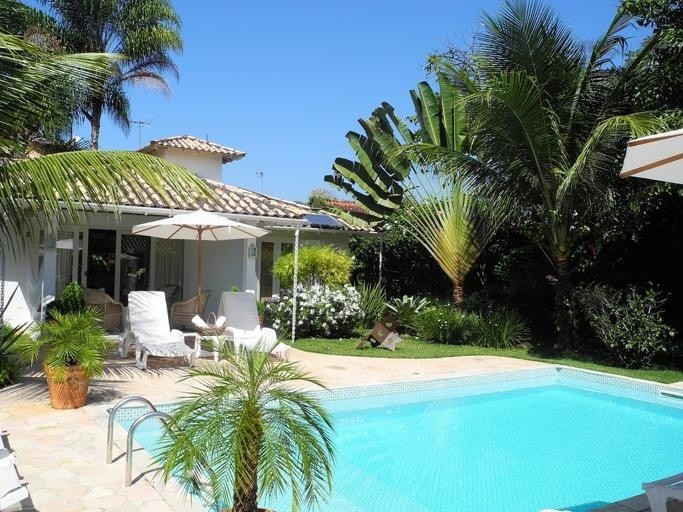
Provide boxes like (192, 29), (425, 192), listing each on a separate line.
(256, 300), (265, 328)
(18, 306), (117, 409)
(151, 330), (339, 512)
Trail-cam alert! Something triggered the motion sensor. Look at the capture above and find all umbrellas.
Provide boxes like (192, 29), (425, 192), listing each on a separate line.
(131, 209), (272, 314)
(620, 128), (682, 185)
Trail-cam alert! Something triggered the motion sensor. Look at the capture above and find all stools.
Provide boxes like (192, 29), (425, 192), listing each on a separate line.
(195, 334), (229, 363)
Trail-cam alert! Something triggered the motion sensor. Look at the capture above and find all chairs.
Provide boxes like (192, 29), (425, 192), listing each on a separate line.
(216, 292), (290, 359)
(118, 290), (192, 372)
(0, 428), (37, 512)
(169, 290), (212, 329)
(154, 284), (179, 315)
(82, 292), (124, 331)
